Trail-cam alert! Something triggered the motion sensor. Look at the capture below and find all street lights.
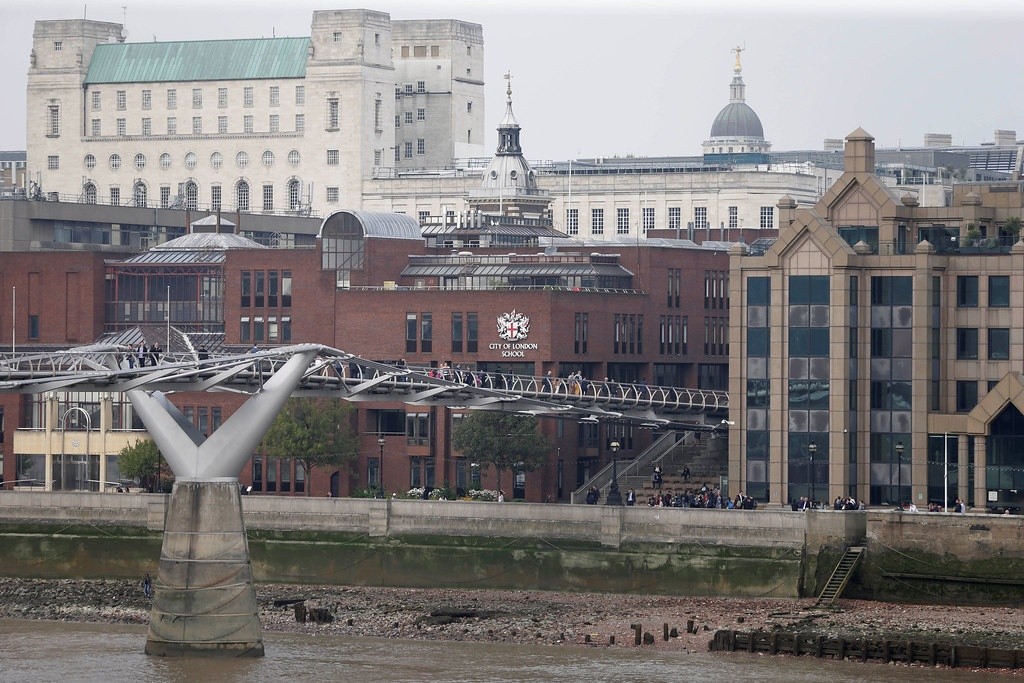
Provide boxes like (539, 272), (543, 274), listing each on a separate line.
(606, 438), (624, 506)
(808, 440), (818, 509)
(896, 443), (906, 512)
(376, 434), (386, 499)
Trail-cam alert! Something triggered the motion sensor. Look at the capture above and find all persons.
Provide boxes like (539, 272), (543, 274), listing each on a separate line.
(454, 364), (462, 383)
(431, 362), (451, 380)
(625, 488), (636, 505)
(398, 358), (407, 382)
(498, 491), (504, 502)
(117, 484), (153, 492)
(508, 368), (513, 390)
(494, 366), (503, 389)
(251, 344), (260, 371)
(632, 378), (648, 398)
(143, 574), (151, 596)
(136, 342), (147, 367)
(125, 344), (136, 369)
(569, 371), (589, 395)
(649, 465), (756, 509)
(694, 421), (701, 444)
(834, 496), (865, 510)
(903, 499), (965, 513)
(335, 354), (365, 378)
(602, 376), (618, 396)
(480, 370), (486, 387)
(115, 347), (123, 370)
(791, 496), (817, 511)
(198, 345), (208, 360)
(545, 371), (552, 390)
(466, 367), (473, 385)
(149, 342), (162, 366)
(587, 485), (600, 505)
